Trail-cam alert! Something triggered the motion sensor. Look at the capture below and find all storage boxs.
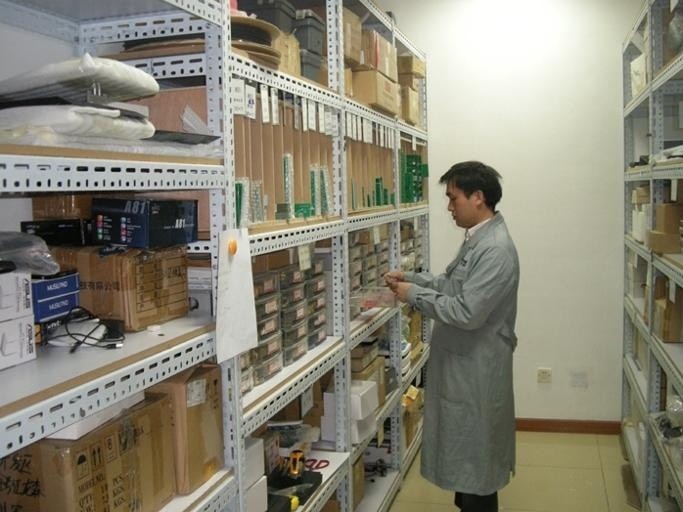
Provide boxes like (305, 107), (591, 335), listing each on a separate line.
(1, 2), (432, 511)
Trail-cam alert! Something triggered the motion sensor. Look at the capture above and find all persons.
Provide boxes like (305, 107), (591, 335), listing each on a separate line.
(382, 160), (522, 512)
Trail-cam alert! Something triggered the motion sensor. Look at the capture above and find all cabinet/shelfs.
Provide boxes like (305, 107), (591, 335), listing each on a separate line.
(621, 0), (683, 512)
